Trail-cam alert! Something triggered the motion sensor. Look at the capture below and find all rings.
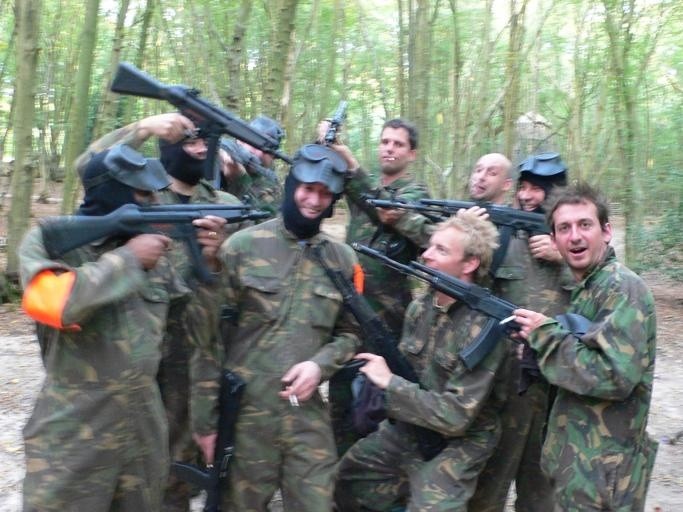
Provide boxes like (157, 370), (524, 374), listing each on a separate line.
(209, 231), (218, 239)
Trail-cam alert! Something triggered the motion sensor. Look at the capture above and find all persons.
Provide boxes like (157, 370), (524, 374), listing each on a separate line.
(510, 186), (656, 512)
(379, 153), (512, 249)
(457, 155), (568, 512)
(333, 218), (509, 511)
(317, 119), (433, 459)
(219, 117), (288, 220)
(18, 146), (229, 512)
(20, 112), (253, 512)
(190, 144), (364, 512)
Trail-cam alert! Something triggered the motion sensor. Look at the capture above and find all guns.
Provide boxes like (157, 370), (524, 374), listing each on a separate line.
(367, 198), (555, 274)
(349, 242), (544, 377)
(172, 371), (245, 511)
(109, 63), (293, 179)
(37, 203), (272, 286)
(323, 103), (349, 139)
(313, 252), (446, 455)
(219, 138), (277, 188)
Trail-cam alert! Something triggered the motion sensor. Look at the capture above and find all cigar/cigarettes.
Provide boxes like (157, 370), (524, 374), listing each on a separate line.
(499, 315), (517, 325)
